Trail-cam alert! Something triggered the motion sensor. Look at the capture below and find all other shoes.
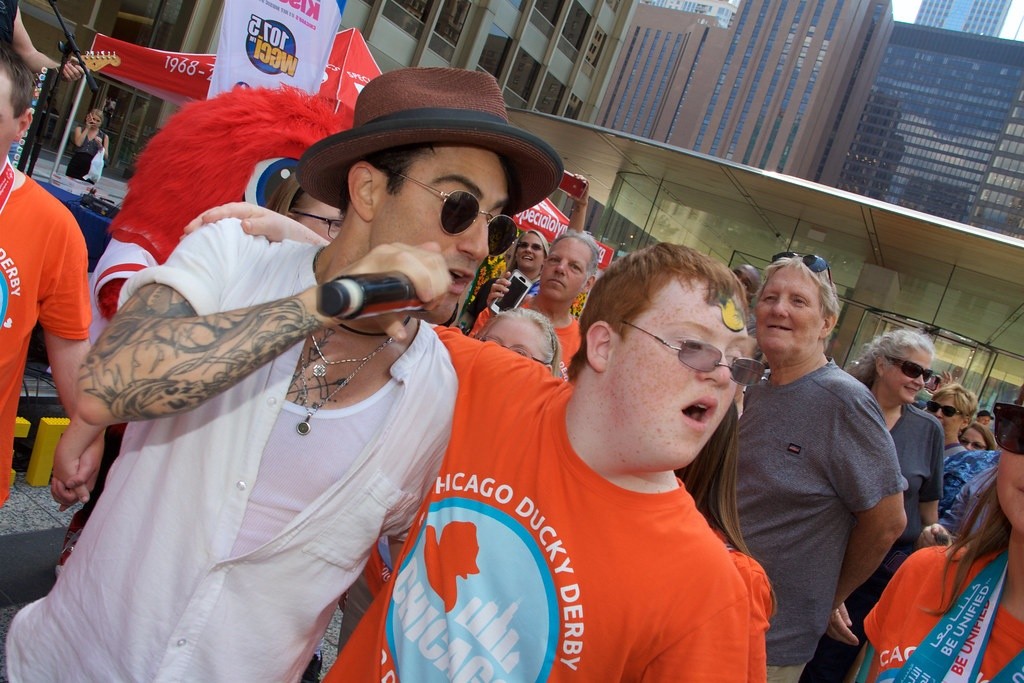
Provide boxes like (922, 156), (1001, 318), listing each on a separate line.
(56, 510), (91, 576)
(300, 652), (322, 683)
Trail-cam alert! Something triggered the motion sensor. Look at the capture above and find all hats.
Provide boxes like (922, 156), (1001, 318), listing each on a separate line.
(295, 67), (564, 217)
(978, 410), (994, 420)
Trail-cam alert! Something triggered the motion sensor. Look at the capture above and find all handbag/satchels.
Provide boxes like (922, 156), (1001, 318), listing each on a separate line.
(83, 147), (105, 183)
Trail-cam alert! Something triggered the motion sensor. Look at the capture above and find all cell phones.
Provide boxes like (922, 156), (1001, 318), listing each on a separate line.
(559, 170), (587, 199)
(489, 269), (533, 314)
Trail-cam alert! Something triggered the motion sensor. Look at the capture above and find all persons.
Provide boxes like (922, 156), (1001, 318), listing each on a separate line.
(0, 0), (1024, 683)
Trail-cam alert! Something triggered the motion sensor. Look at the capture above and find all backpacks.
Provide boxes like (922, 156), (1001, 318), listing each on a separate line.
(102, 133), (112, 167)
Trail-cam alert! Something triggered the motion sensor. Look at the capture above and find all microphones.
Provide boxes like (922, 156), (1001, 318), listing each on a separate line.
(315, 269), (426, 320)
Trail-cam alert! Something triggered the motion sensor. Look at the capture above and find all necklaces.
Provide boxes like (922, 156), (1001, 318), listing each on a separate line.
(310, 319), (386, 379)
(312, 247), (410, 336)
(295, 317), (396, 436)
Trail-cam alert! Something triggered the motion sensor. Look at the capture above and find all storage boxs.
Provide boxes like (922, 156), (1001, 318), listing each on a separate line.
(52, 172), (91, 196)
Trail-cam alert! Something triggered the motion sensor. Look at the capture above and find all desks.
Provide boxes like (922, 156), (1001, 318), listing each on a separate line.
(33, 179), (116, 231)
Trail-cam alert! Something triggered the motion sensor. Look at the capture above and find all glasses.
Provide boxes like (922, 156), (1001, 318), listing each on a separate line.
(392, 171), (519, 256)
(479, 335), (550, 365)
(926, 400), (969, 417)
(960, 439), (986, 450)
(292, 210), (344, 239)
(517, 241), (543, 251)
(771, 252), (831, 284)
(885, 355), (934, 382)
(622, 320), (766, 386)
(993, 403), (1024, 454)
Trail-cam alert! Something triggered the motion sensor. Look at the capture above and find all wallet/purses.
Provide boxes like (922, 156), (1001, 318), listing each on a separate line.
(884, 551), (909, 575)
